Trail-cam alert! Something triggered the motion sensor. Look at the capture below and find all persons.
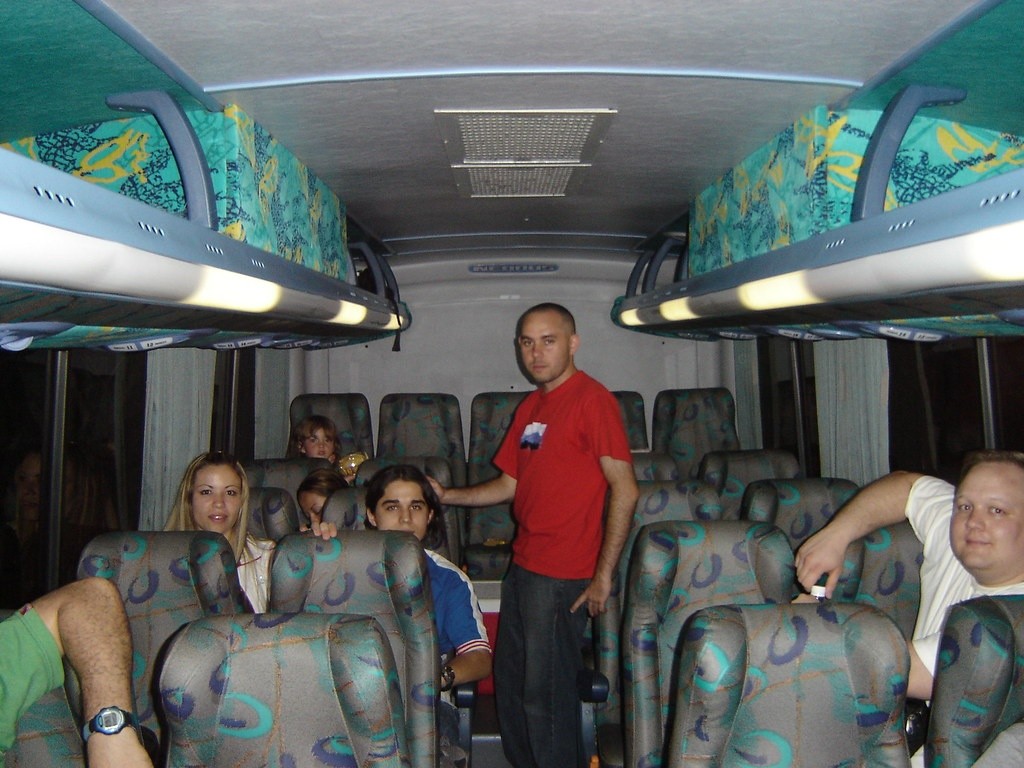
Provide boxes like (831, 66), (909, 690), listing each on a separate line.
(365, 462), (493, 746)
(0, 576), (153, 768)
(427, 302), (639, 768)
(791, 447), (1024, 768)
(163, 449), (337, 614)
(292, 414), (359, 523)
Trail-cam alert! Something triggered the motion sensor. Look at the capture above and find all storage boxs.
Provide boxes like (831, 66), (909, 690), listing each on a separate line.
(0, 104), (356, 349)
(687, 104), (1024, 335)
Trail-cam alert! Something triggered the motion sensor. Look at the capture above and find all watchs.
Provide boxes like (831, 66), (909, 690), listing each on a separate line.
(83, 706), (138, 742)
(440, 665), (455, 692)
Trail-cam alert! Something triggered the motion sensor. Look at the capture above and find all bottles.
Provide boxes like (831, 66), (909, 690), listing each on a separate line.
(810, 585), (827, 603)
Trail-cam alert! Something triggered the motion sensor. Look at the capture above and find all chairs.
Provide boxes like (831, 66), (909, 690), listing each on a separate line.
(579, 449), (1024, 768)
(284, 386), (743, 598)
(1, 456), (478, 768)
